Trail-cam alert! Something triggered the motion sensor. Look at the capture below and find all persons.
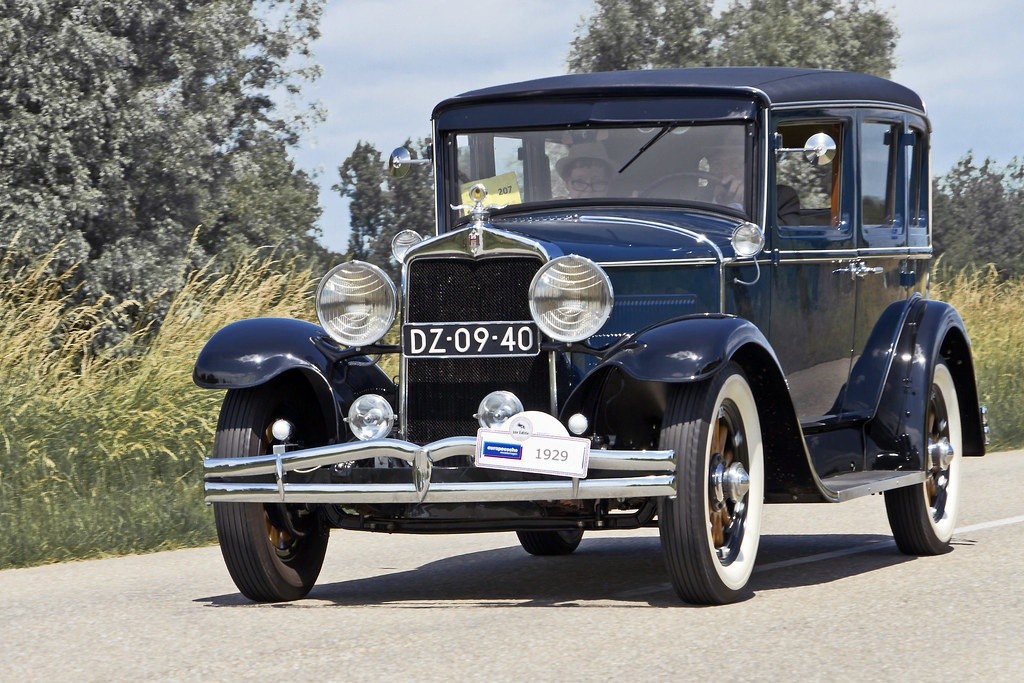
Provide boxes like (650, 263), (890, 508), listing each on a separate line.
(693, 127), (800, 226)
(554, 142), (623, 198)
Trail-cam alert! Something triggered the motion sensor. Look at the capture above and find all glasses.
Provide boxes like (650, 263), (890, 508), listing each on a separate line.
(569, 178), (609, 193)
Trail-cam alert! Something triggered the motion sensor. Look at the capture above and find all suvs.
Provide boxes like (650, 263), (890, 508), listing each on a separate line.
(191, 57), (987, 605)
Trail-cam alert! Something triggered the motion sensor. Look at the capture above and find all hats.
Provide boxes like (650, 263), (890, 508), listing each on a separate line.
(555, 142), (617, 181)
